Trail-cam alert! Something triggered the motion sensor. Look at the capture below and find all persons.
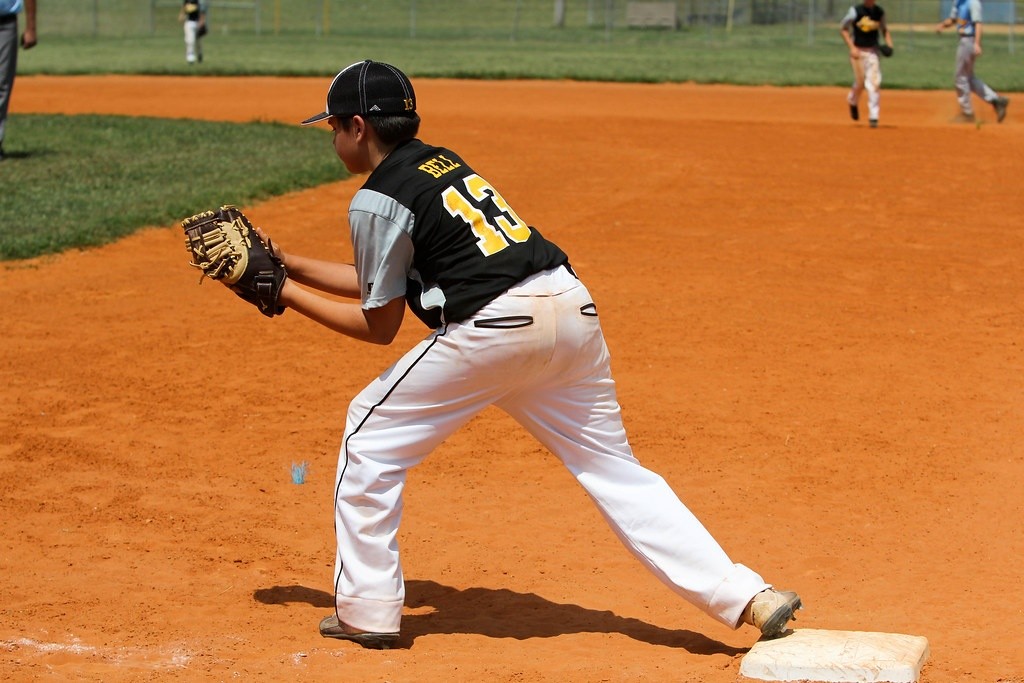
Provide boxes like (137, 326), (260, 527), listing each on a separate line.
(839, 0), (893, 128)
(177, 0), (209, 68)
(932, 0), (1009, 126)
(181, 57), (800, 648)
(0, 0), (36, 162)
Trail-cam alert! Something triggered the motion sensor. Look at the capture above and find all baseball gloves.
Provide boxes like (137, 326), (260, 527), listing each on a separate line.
(877, 45), (893, 57)
(197, 25), (207, 38)
(179, 203), (290, 318)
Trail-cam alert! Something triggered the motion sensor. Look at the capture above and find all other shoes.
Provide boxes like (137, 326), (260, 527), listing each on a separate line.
(850, 105), (858, 120)
(870, 120), (877, 127)
(992, 96), (1007, 121)
(950, 112), (975, 122)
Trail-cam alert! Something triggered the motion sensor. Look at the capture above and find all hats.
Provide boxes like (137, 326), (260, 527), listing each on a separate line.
(301, 59), (416, 125)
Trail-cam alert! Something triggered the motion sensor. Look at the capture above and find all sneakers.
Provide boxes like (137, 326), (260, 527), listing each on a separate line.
(740, 587), (802, 637)
(319, 612), (400, 640)
